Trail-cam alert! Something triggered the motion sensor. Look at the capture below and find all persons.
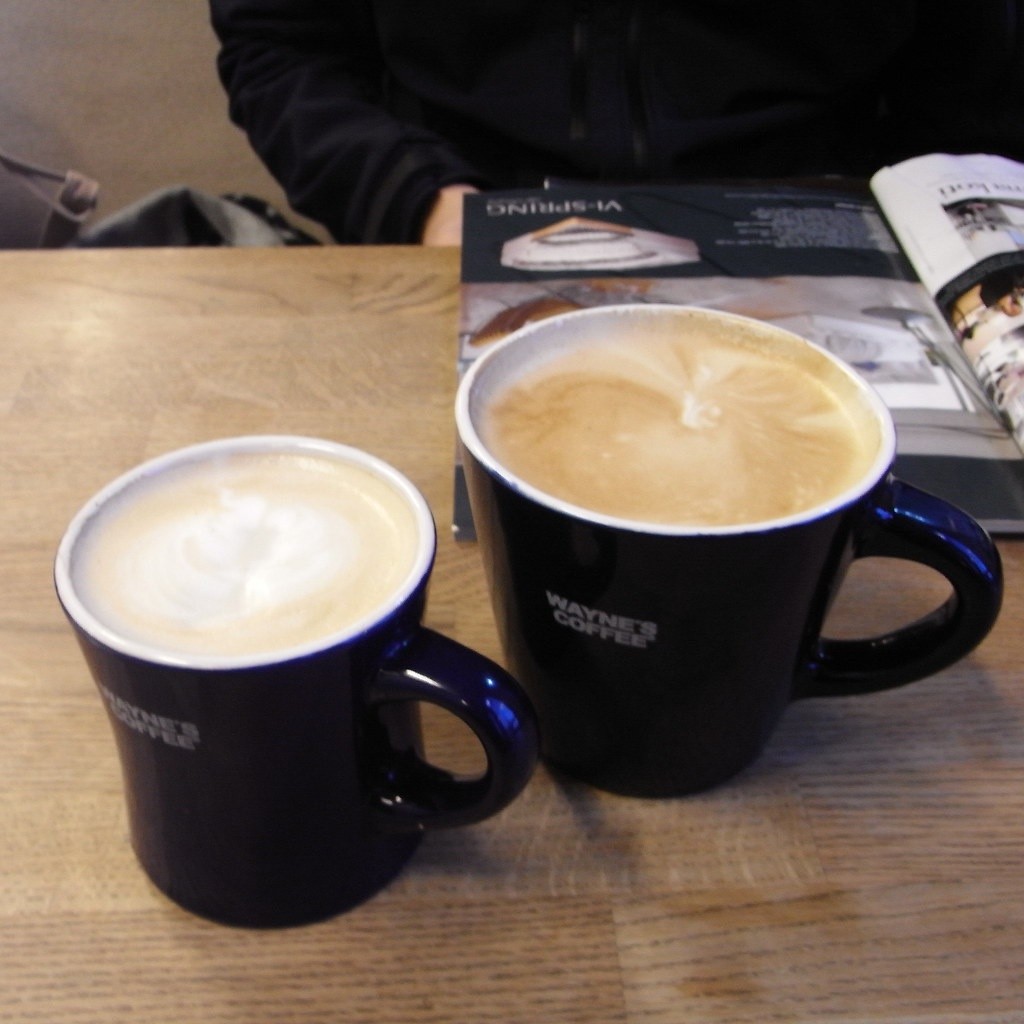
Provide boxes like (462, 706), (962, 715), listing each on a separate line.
(206, 0), (1024, 251)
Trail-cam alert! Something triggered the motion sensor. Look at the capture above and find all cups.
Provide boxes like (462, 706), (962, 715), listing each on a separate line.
(54, 435), (538, 927)
(458, 304), (1004, 796)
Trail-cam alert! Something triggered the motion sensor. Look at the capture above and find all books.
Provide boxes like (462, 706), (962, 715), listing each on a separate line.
(450, 147), (1023, 551)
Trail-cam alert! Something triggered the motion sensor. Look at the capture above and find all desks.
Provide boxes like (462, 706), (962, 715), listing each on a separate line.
(0, 243), (1023, 1024)
